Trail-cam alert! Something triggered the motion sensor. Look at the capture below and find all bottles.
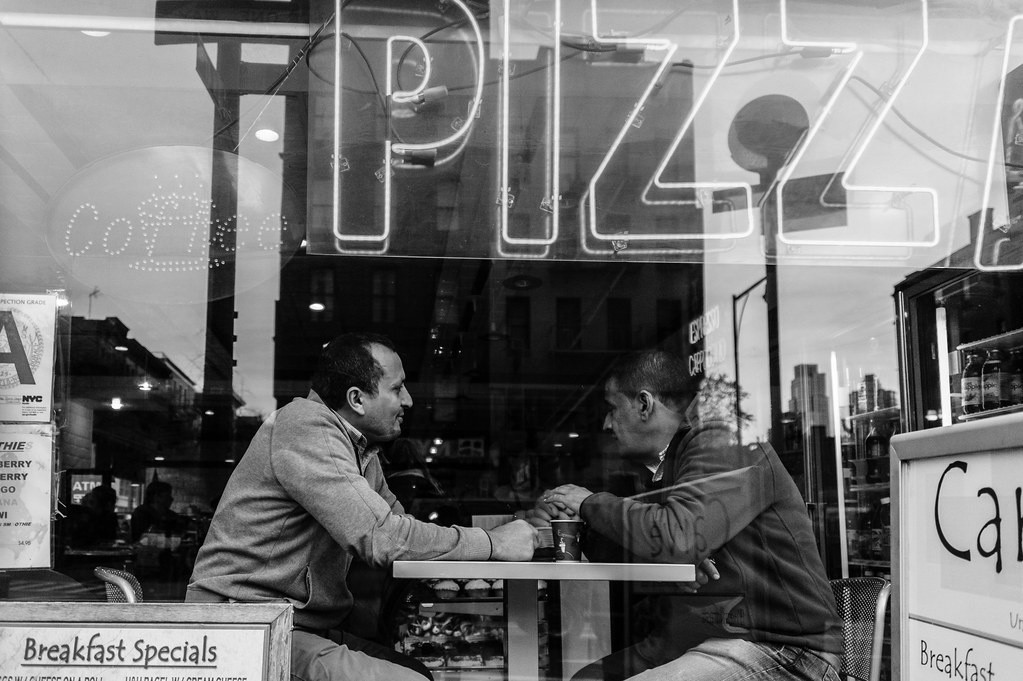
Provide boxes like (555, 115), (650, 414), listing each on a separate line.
(961, 344), (1023, 414)
(840, 340), (899, 561)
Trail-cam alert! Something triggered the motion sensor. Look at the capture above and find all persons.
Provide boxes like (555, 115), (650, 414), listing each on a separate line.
(130, 481), (198, 583)
(541, 347), (842, 681)
(381, 436), (445, 513)
(184, 330), (540, 681)
(69, 485), (121, 551)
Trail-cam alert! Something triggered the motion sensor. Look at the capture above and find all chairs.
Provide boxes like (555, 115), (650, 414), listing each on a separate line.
(93, 566), (143, 603)
(830, 577), (893, 681)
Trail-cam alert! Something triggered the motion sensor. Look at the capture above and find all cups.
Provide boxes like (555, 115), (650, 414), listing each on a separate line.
(536, 526), (556, 561)
(551, 518), (585, 563)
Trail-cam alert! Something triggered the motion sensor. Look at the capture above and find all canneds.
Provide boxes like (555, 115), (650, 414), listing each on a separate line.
(961, 348), (1023, 413)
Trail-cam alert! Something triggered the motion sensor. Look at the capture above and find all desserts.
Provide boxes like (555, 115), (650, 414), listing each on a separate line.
(403, 579), (551, 666)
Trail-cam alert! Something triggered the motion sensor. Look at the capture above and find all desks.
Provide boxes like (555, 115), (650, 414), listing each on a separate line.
(389, 560), (696, 681)
(63, 546), (162, 600)
